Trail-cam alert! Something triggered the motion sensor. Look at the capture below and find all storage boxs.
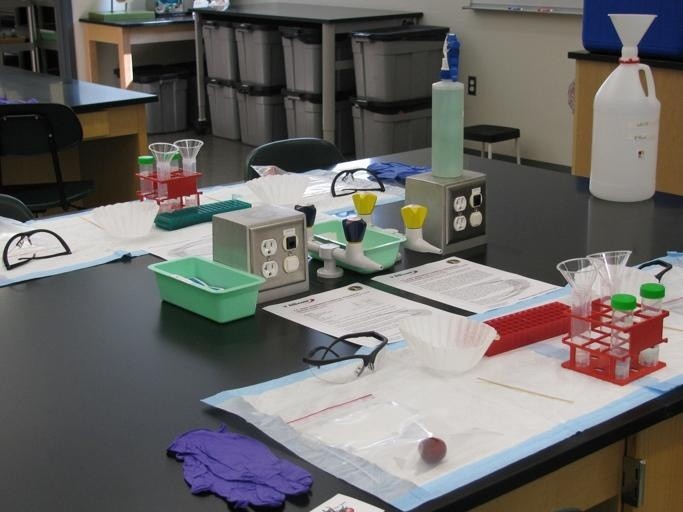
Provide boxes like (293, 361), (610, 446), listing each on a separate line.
(111, 63), (193, 137)
(307, 215), (407, 276)
(196, 12), (450, 165)
(142, 253), (267, 323)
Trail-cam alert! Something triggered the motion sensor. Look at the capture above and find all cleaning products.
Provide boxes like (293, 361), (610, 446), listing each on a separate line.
(431, 33), (466, 179)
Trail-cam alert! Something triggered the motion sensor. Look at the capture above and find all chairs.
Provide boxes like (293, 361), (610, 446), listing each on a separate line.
(0, 192), (33, 223)
(243, 135), (346, 182)
(0, 102), (97, 217)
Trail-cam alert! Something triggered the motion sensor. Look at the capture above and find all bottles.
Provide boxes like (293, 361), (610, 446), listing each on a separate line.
(600, 283), (623, 350)
(637, 282), (665, 367)
(182, 160), (198, 210)
(137, 155), (155, 202)
(609, 294), (637, 379)
(571, 288), (592, 366)
(154, 163), (171, 208)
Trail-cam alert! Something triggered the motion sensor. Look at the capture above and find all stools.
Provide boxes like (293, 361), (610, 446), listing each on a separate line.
(463, 123), (520, 167)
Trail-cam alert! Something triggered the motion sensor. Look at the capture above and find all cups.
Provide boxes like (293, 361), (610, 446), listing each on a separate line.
(162, 151), (181, 172)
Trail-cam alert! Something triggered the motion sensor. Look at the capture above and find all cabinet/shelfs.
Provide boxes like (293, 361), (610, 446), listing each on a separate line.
(0, 0), (62, 77)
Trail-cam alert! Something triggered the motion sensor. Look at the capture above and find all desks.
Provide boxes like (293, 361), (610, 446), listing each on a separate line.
(566, 45), (682, 197)
(1, 64), (158, 213)
(77, 12), (209, 90)
(188, 2), (424, 162)
(0, 139), (683, 512)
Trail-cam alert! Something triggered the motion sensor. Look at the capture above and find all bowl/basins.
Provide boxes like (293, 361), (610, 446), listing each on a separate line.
(397, 314), (498, 376)
